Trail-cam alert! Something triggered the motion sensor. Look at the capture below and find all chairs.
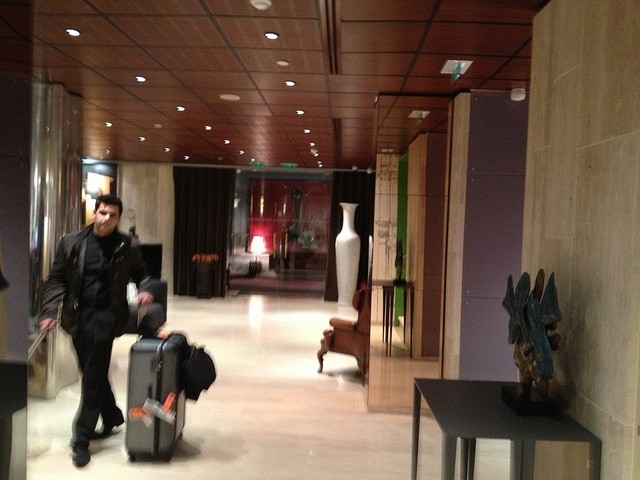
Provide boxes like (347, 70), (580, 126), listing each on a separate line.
(317, 288), (370, 387)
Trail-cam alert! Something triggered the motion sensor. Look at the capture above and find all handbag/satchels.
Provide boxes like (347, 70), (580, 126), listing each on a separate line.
(27, 326), (79, 399)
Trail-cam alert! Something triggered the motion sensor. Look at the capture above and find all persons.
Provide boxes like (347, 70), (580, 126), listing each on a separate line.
(36, 194), (157, 467)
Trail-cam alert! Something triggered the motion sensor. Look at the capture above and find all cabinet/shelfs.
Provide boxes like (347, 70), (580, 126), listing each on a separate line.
(131, 244), (162, 289)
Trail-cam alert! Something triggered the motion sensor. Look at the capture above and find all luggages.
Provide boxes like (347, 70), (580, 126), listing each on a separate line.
(125, 301), (187, 463)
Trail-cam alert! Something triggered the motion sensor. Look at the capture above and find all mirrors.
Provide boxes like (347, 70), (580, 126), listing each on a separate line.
(226, 169), (333, 299)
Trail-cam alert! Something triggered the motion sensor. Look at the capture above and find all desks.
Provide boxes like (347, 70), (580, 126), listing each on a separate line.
(371, 280), (395, 358)
(411, 378), (606, 480)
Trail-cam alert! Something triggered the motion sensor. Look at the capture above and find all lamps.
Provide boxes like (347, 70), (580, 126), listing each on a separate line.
(250, 235), (263, 278)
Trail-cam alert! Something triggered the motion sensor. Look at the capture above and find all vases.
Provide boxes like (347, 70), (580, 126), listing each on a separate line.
(335, 202), (362, 302)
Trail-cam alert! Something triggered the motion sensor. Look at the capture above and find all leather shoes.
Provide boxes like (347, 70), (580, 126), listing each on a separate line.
(72, 442), (90, 467)
(90, 406), (124, 439)
